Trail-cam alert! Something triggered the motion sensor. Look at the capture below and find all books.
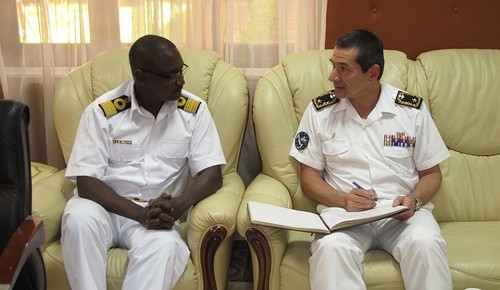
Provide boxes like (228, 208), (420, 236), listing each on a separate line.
(247, 201), (409, 234)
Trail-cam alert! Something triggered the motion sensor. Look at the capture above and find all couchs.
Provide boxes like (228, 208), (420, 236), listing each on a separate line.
(235, 49), (500, 290)
(30, 46), (251, 290)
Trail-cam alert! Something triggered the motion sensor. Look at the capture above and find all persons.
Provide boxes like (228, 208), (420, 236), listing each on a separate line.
(60, 35), (227, 290)
(290, 29), (452, 290)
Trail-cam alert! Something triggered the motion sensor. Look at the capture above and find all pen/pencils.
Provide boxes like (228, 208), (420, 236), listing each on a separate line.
(353, 181), (382, 204)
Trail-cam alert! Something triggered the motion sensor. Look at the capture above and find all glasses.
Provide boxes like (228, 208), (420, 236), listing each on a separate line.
(142, 60), (189, 83)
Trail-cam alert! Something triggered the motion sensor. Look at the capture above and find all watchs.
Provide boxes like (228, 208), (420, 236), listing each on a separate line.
(408, 193), (422, 210)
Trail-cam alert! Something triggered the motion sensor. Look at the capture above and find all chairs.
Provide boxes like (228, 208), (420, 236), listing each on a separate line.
(0, 99), (48, 290)
